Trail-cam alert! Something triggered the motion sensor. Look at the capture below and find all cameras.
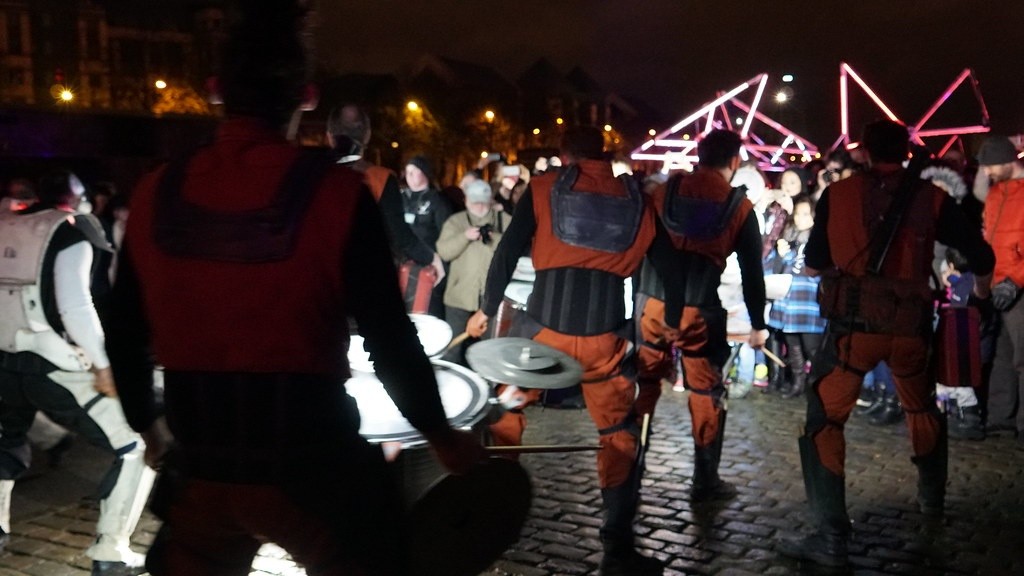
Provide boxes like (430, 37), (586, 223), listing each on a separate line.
(479, 223), (494, 243)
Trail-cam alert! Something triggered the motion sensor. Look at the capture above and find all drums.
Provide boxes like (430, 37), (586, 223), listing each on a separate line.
(340, 357), (503, 500)
(342, 311), (453, 371)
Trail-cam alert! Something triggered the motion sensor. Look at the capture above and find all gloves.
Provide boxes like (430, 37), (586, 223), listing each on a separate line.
(994, 279), (1016, 310)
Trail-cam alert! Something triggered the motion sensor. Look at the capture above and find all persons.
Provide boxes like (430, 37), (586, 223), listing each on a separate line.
(101, 9), (500, 576)
(776, 122), (995, 560)
(908, 133), (1023, 439)
(632, 128), (773, 502)
(0, 164), (157, 576)
(710, 150), (904, 429)
(298, 101), (534, 364)
(465, 124), (667, 576)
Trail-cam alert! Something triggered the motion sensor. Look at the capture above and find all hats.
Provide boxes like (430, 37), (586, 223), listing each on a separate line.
(466, 181), (492, 205)
(978, 136), (1017, 164)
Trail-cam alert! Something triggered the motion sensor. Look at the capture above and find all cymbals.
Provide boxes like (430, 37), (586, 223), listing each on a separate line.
(466, 337), (583, 389)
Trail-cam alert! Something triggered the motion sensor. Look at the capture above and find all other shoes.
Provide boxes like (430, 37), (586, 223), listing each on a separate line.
(692, 479), (735, 502)
(761, 382), (988, 440)
(916, 488), (944, 515)
(93, 560), (148, 576)
(600, 551), (665, 576)
(777, 531), (847, 567)
